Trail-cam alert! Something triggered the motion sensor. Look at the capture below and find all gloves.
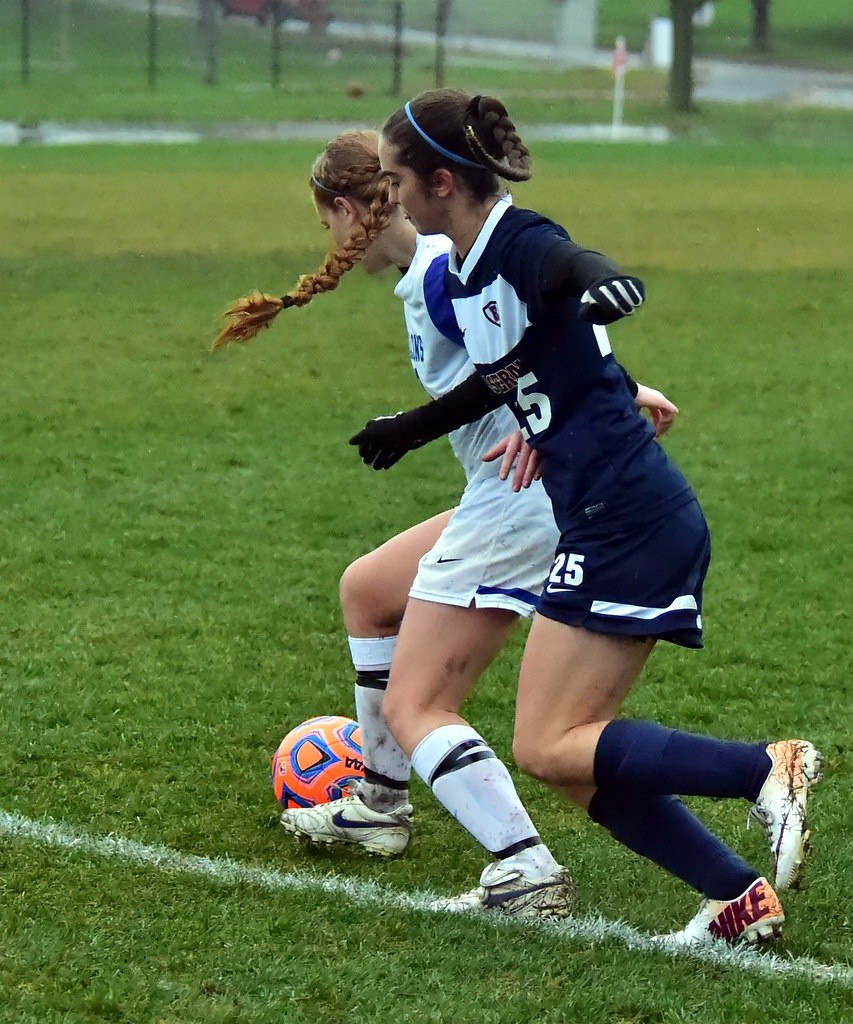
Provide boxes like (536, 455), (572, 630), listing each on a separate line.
(547, 247), (645, 325)
(348, 406), (436, 470)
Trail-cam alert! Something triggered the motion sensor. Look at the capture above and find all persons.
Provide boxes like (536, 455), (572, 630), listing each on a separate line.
(210, 86), (818, 951)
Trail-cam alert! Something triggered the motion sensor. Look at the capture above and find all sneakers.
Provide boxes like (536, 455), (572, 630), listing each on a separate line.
(280, 779), (414, 862)
(649, 876), (785, 955)
(429, 861), (574, 925)
(747, 739), (826, 894)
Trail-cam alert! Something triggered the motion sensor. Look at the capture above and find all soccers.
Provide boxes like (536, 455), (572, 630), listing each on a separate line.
(273, 714), (365, 813)
